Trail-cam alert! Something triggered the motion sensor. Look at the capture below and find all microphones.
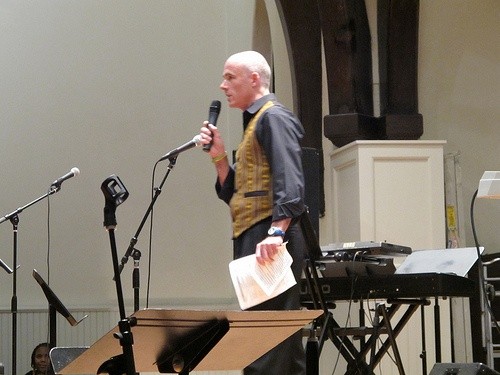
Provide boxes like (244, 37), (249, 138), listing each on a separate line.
(51, 167), (80, 186)
(202, 99), (221, 151)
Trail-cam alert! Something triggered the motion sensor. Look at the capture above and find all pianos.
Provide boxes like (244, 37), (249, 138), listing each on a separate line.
(298, 271), (477, 375)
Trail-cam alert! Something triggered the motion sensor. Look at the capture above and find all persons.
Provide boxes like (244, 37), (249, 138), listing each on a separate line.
(200, 51), (307, 375)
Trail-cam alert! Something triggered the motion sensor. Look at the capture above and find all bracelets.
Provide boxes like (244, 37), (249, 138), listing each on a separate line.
(211, 151), (227, 164)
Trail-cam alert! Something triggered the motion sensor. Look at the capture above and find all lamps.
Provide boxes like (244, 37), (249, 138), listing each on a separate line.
(471, 171), (500, 331)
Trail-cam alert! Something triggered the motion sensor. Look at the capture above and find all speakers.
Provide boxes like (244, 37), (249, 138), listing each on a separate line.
(429, 363), (500, 375)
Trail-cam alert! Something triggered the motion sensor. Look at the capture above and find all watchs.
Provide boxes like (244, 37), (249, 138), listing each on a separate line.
(268, 227), (285, 237)
